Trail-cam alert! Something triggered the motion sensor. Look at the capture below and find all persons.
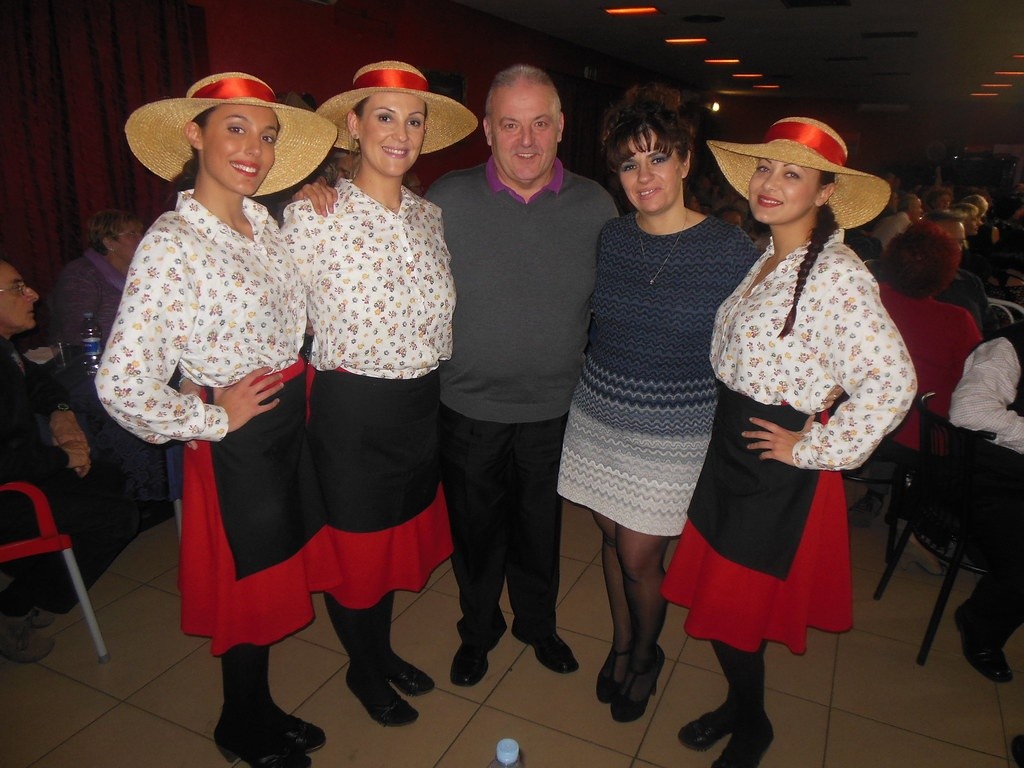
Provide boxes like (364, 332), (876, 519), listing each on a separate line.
(604, 171), (635, 217)
(51, 209), (144, 346)
(685, 172), (772, 255)
(422, 65), (619, 688)
(270, 151), (359, 228)
(556, 82), (759, 723)
(0, 259), (90, 663)
(846, 167), (1024, 575)
(179, 60), (477, 727)
(662, 116), (918, 768)
(948, 321), (1024, 683)
(404, 171), (424, 197)
(93, 72), (337, 768)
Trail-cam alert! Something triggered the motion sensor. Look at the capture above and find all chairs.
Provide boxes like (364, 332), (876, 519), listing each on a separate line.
(0, 481), (110, 663)
(835, 264), (1023, 666)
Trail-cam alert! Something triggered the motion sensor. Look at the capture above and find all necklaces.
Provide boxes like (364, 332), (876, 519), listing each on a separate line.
(636, 212), (685, 286)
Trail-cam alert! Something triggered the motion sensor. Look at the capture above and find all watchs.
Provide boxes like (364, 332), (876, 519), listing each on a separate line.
(50, 402), (69, 411)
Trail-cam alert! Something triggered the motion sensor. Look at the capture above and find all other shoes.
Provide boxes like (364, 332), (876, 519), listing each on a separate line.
(346, 669), (420, 726)
(679, 709), (740, 751)
(897, 464), (921, 521)
(383, 655), (436, 696)
(1011, 735), (1024, 768)
(711, 721), (773, 768)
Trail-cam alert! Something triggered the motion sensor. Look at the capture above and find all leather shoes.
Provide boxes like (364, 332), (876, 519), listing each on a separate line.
(955, 601), (1013, 682)
(450, 636), (497, 686)
(511, 618), (579, 674)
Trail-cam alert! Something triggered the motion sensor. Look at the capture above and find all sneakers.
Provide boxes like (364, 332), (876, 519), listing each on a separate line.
(14, 607), (53, 628)
(0, 615), (54, 663)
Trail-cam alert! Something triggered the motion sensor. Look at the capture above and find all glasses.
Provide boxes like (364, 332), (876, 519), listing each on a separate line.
(0, 282), (26, 298)
(101, 230), (143, 239)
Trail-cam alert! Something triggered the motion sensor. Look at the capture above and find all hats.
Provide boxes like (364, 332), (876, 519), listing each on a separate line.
(707, 117), (891, 231)
(126, 71), (338, 198)
(313, 60), (478, 156)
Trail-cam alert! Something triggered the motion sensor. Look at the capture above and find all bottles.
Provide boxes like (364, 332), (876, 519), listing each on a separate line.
(486, 738), (522, 768)
(80, 311), (102, 379)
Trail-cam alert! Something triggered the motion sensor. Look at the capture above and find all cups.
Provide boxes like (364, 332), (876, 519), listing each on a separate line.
(50, 341), (73, 368)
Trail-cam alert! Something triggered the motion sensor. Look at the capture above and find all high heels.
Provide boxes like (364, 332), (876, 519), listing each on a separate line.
(610, 642), (665, 722)
(247, 702), (326, 752)
(213, 722), (312, 768)
(597, 637), (634, 703)
